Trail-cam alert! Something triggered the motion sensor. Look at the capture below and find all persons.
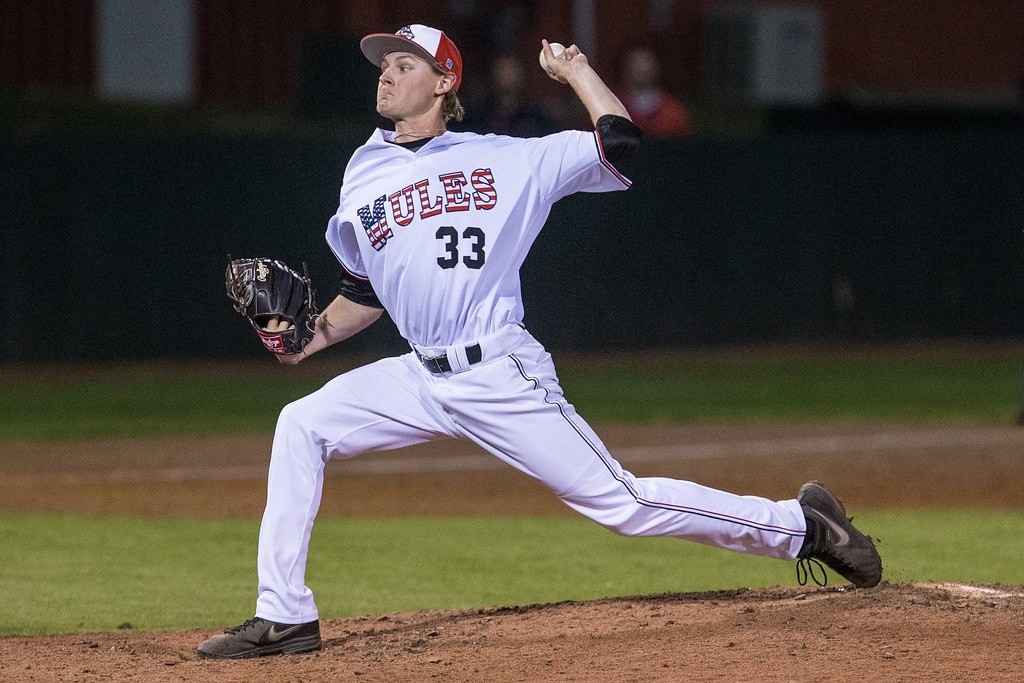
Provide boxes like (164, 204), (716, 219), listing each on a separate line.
(198, 24), (882, 662)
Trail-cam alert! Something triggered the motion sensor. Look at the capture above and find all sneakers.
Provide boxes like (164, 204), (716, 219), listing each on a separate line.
(195, 617), (322, 659)
(796, 480), (882, 588)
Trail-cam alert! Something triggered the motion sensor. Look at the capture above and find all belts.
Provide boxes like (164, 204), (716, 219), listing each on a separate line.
(413, 344), (482, 373)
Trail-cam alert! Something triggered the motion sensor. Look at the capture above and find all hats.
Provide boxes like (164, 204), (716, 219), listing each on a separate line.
(359, 24), (462, 93)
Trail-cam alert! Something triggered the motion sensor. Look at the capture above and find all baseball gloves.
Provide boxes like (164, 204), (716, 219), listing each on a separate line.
(225, 252), (325, 356)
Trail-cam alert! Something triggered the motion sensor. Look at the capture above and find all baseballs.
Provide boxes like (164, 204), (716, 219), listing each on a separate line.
(538, 43), (567, 74)
